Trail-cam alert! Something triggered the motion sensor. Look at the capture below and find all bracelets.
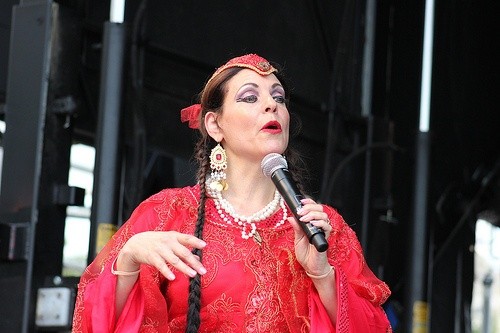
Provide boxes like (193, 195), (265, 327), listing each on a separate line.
(305, 266), (333, 280)
(111, 255), (142, 275)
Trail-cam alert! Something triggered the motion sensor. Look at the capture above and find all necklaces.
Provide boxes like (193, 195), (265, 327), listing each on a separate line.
(210, 190), (287, 240)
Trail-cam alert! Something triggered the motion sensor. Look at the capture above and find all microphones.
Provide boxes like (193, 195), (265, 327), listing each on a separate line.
(261, 153), (329, 253)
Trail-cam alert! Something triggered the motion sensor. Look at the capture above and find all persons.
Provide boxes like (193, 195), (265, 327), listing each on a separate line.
(72, 54), (395, 333)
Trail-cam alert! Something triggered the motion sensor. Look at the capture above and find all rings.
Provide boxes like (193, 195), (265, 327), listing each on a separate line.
(327, 218), (331, 225)
